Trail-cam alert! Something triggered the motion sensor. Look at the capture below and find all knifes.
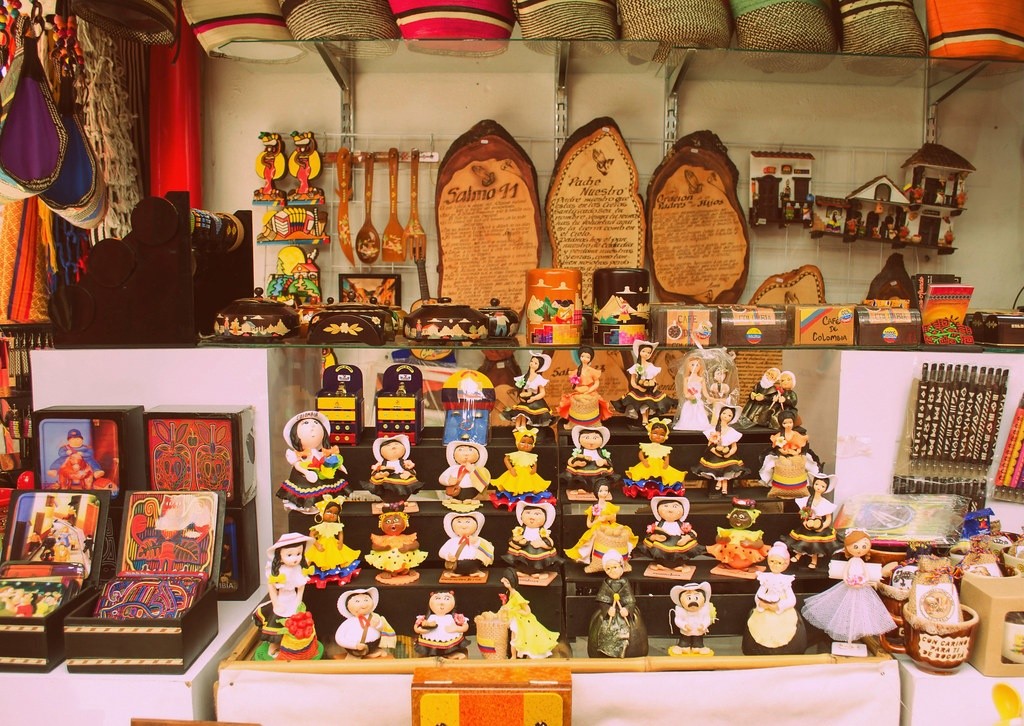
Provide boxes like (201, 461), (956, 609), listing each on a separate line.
(336, 147), (355, 267)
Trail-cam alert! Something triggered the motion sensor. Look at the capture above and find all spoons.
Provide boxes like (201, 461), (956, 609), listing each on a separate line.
(355, 155), (380, 264)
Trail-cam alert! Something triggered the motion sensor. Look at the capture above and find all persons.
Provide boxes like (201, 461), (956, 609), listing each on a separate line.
(560, 402), (898, 657)
(556, 345), (614, 429)
(501, 353), (551, 428)
(611, 340), (679, 424)
(264, 410), (557, 658)
(742, 367), (803, 430)
(674, 356), (743, 431)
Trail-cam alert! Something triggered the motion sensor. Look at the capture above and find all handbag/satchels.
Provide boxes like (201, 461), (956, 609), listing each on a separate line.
(0, 13), (108, 230)
(53, 0), (1024, 80)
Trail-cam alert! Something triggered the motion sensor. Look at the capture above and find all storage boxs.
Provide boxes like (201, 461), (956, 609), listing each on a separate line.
(32, 405), (261, 602)
(960, 578), (1024, 677)
(0, 489), (112, 674)
(62, 490), (227, 675)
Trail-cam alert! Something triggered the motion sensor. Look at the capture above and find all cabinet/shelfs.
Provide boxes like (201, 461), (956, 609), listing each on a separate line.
(195, 38), (1024, 354)
(781, 348), (1023, 726)
(557, 416), (843, 643)
(0, 346), (321, 726)
(290, 426), (563, 641)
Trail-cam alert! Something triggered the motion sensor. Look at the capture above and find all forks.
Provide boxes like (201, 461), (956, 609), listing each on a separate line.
(402, 148), (427, 261)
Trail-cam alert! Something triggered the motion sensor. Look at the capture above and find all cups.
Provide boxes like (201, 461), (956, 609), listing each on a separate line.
(879, 603), (980, 675)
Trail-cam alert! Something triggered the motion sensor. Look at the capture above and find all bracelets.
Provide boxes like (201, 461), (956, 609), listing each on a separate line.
(191, 208), (244, 251)
